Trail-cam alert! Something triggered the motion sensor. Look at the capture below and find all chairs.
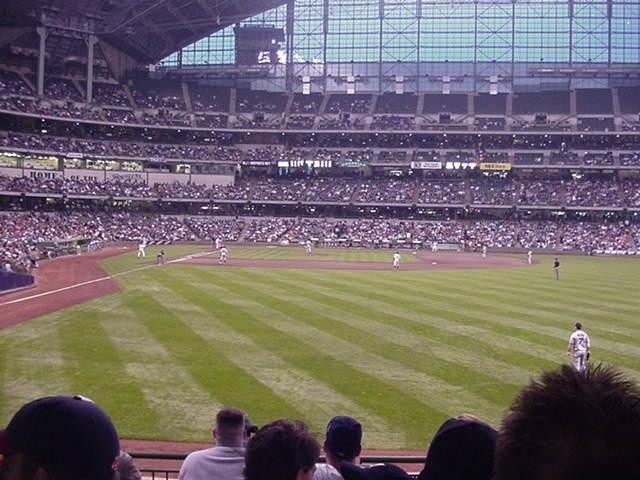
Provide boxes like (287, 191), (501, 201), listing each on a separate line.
(0, 64), (639, 158)
(0, 158), (640, 290)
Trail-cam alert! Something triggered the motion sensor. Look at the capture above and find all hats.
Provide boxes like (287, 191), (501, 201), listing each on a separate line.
(325, 416), (363, 458)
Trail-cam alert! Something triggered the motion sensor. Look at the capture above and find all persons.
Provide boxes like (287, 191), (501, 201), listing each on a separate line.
(339, 460), (409, 480)
(242, 419), (320, 480)
(567, 322), (590, 371)
(1, 71), (639, 275)
(552, 257), (560, 281)
(312, 414), (386, 480)
(177, 406), (251, 480)
(492, 361), (640, 480)
